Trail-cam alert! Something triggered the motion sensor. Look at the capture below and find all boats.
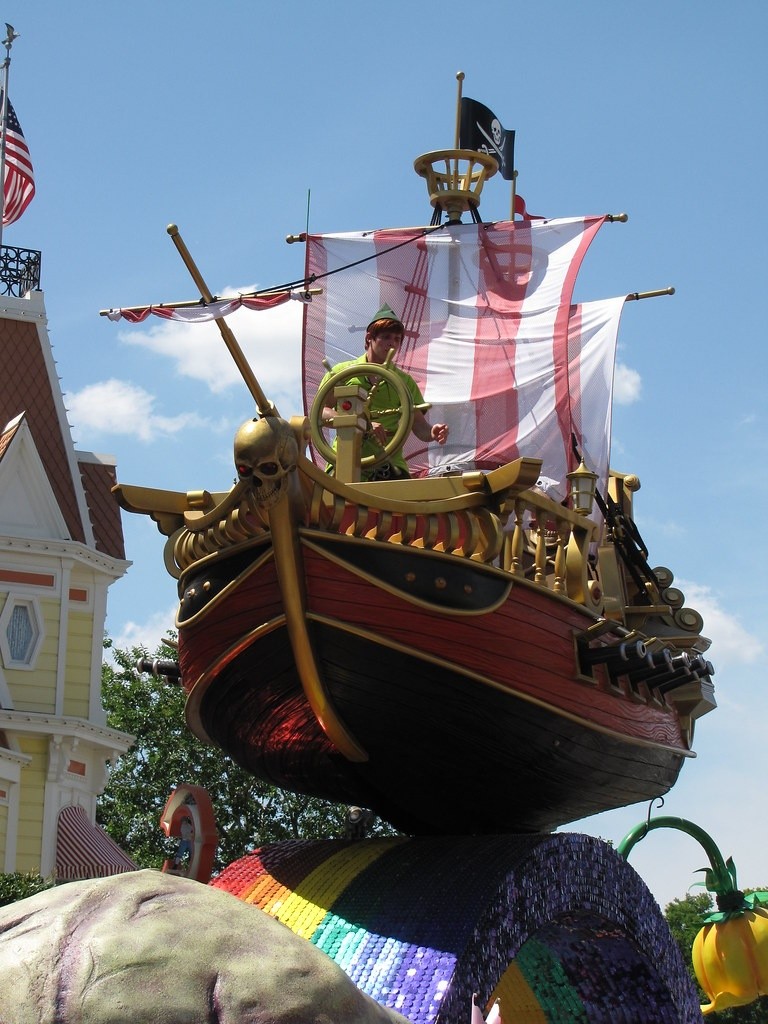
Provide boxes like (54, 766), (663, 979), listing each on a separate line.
(100, 70), (716, 838)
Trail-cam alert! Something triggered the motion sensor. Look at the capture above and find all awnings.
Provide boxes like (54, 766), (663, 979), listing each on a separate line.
(56, 806), (139, 878)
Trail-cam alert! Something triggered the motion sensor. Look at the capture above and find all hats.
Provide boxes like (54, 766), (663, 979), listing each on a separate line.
(367, 301), (400, 330)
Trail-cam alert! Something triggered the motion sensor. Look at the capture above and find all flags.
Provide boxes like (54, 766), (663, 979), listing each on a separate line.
(0, 87), (35, 227)
(459, 97), (516, 180)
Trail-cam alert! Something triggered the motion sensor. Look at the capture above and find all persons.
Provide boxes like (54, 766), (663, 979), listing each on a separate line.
(172, 816), (194, 870)
(317, 301), (449, 483)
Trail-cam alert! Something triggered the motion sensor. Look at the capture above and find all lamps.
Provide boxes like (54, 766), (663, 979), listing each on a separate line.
(565, 455), (599, 517)
